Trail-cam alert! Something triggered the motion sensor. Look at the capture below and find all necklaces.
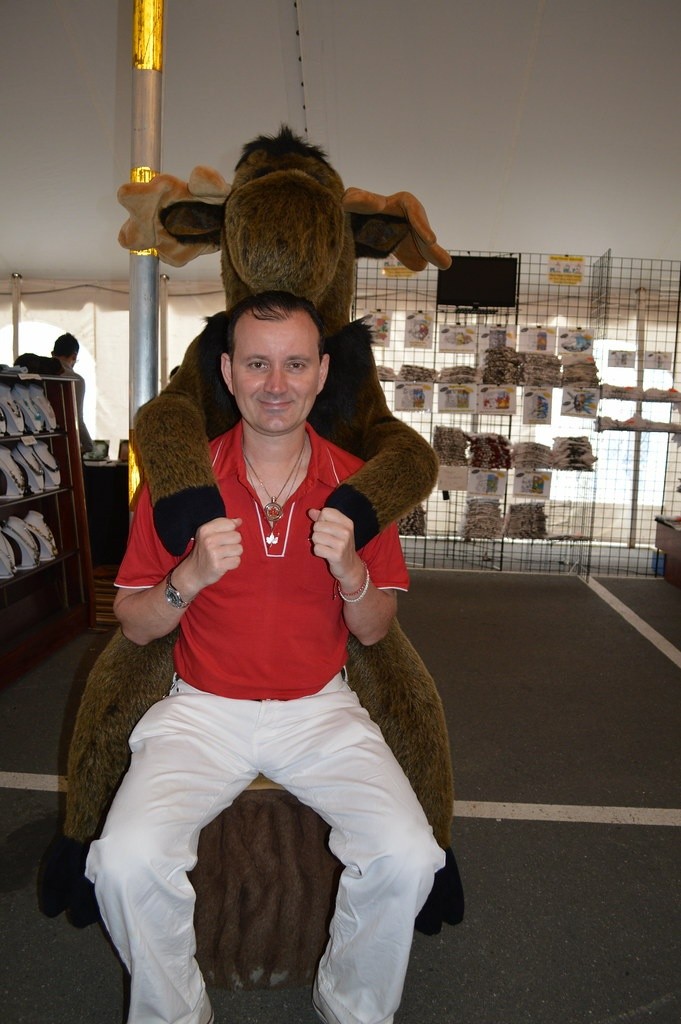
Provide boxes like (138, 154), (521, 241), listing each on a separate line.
(242, 439), (307, 549)
(0, 390), (61, 573)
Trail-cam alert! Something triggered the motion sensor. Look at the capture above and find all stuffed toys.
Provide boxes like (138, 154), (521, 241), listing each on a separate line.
(38, 125), (465, 935)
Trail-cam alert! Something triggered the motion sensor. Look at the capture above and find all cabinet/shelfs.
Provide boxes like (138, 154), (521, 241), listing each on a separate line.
(0, 373), (95, 695)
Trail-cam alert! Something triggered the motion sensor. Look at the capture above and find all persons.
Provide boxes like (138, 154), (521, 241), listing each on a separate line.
(52, 332), (94, 453)
(85, 289), (446, 1024)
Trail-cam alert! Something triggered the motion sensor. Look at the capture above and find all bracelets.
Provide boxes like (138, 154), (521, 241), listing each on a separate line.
(331, 560), (370, 603)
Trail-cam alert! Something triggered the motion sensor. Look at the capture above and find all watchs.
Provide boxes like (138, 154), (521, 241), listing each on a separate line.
(165, 568), (191, 609)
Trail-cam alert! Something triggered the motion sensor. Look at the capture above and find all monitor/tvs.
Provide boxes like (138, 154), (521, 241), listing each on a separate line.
(437, 256), (517, 307)
